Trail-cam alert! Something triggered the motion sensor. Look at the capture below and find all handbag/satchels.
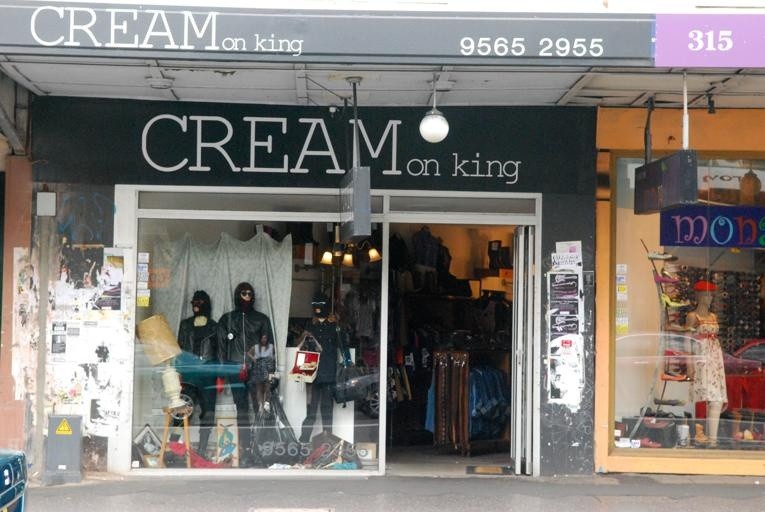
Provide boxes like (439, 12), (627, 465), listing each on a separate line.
(287, 335), (323, 384)
(334, 361), (368, 408)
(250, 390), (300, 467)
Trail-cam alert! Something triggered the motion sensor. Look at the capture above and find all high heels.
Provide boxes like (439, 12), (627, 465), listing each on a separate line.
(736, 429), (765, 440)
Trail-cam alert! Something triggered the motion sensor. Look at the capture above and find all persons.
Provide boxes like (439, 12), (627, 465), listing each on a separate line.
(163, 289), (226, 461)
(684, 281), (729, 450)
(299, 290), (344, 444)
(216, 282), (279, 468)
(249, 332), (274, 411)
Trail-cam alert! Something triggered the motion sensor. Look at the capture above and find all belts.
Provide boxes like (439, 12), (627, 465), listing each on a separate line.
(436, 353), (471, 457)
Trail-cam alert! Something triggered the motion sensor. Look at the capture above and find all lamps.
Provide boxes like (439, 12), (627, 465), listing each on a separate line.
(419, 74), (449, 144)
(319, 221), (381, 267)
(135, 313), (189, 410)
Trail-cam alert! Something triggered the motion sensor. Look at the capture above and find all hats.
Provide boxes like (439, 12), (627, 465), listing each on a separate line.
(694, 281), (716, 292)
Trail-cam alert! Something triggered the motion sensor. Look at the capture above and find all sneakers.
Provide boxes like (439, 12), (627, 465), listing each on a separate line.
(641, 438), (662, 449)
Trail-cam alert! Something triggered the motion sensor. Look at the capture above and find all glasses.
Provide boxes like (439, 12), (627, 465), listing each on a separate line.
(241, 292), (252, 297)
(679, 266), (762, 352)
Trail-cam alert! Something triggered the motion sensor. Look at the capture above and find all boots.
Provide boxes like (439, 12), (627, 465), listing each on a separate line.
(695, 424), (709, 441)
